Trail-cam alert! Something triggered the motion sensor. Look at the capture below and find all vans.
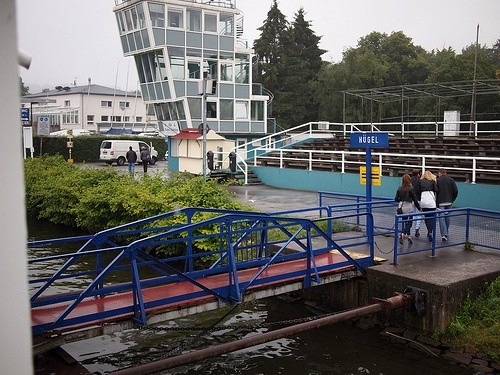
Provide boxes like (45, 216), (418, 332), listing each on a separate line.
(98, 139), (159, 167)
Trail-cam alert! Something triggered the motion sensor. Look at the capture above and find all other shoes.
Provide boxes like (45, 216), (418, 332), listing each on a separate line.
(415, 229), (420, 237)
(399, 234), (403, 245)
(407, 237), (415, 244)
(427, 232), (433, 242)
(442, 235), (448, 241)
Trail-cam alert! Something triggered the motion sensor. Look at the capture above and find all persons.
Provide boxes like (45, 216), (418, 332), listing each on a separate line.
(141, 146), (150, 172)
(395, 174), (422, 245)
(417, 171), (437, 240)
(126, 146), (137, 175)
(409, 169), (422, 237)
(433, 168), (458, 241)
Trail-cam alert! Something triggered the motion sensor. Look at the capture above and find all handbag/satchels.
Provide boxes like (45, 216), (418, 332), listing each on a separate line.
(396, 208), (403, 215)
